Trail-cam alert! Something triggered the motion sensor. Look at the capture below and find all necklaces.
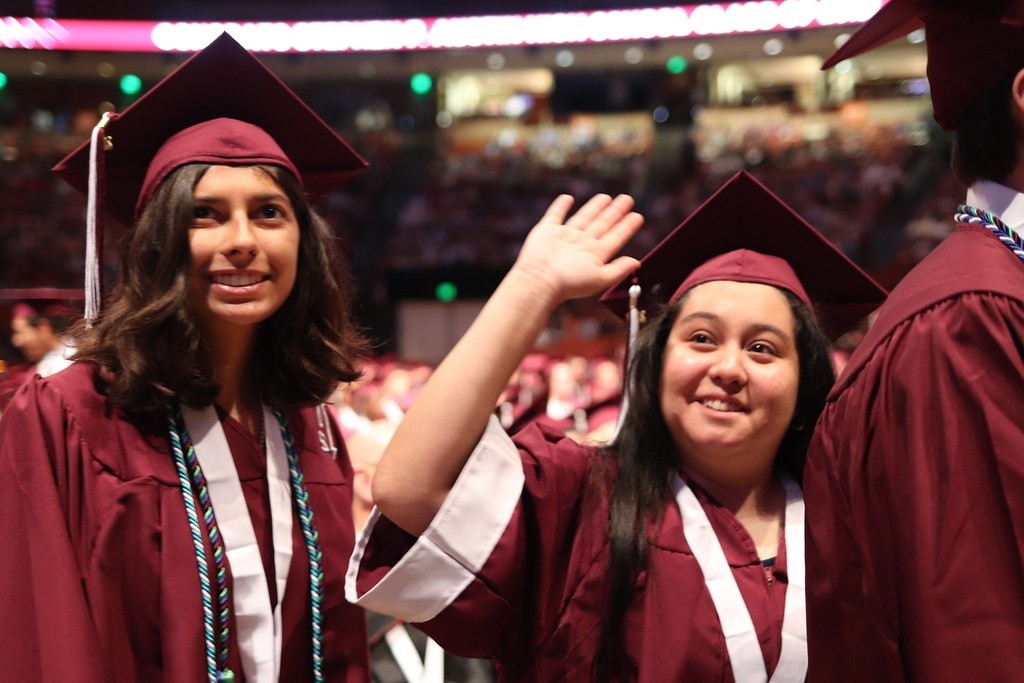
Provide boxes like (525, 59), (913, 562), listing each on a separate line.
(164, 401), (325, 683)
(952, 206), (1024, 261)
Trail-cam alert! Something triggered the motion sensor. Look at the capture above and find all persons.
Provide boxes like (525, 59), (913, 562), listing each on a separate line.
(343, 169), (892, 682)
(1, 33), (371, 682)
(803, 0), (1024, 683)
(2, 298), (862, 523)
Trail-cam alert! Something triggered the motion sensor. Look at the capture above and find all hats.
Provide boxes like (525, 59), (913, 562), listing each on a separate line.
(821, 1), (1023, 132)
(50, 29), (371, 331)
(5, 286), (84, 321)
(566, 170), (889, 456)
